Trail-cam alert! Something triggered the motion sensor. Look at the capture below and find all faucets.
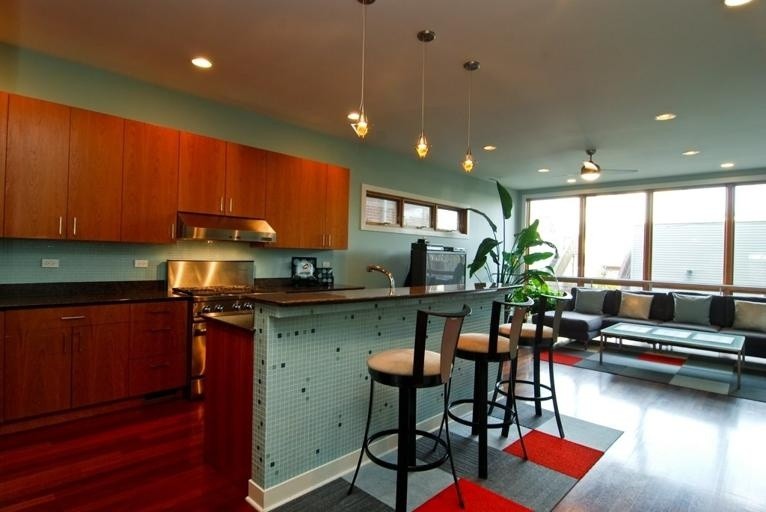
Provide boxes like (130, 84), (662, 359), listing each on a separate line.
(366, 263), (396, 288)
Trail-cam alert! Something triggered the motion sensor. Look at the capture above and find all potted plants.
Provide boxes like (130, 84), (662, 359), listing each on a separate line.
(466, 181), (562, 324)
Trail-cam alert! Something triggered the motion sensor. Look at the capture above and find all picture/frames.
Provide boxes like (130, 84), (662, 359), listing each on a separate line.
(291, 257), (317, 288)
(345, 306), (466, 512)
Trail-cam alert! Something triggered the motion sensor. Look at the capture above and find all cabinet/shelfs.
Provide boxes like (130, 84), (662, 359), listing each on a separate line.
(123, 119), (227, 249)
(300, 158), (349, 250)
(0, 299), (189, 434)
(1, 91), (122, 246)
(226, 141), (301, 250)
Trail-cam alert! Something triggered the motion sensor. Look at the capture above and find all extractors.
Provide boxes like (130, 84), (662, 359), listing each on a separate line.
(176, 212), (277, 244)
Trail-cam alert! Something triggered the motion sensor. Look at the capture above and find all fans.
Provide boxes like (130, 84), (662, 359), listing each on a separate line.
(553, 149), (639, 178)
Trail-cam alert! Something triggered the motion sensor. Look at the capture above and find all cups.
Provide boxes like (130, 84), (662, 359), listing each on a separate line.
(313, 267), (333, 286)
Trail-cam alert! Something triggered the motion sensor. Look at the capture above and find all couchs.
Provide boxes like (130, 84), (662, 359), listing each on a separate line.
(534, 285), (766, 355)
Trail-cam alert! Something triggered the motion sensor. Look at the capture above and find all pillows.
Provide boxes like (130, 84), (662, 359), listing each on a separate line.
(618, 292), (655, 321)
(574, 290), (608, 314)
(671, 292), (712, 325)
(732, 300), (766, 329)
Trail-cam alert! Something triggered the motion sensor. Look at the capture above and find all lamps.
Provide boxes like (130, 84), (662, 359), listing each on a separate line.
(581, 171), (599, 184)
(415, 28), (434, 159)
(462, 59), (478, 174)
(348, 0), (373, 138)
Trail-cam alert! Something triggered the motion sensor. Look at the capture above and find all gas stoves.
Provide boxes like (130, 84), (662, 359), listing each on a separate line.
(172, 282), (288, 318)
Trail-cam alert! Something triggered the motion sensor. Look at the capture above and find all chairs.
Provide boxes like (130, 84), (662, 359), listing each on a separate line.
(487, 291), (574, 439)
(429, 297), (534, 480)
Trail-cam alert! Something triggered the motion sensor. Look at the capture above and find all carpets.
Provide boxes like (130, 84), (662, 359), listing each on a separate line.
(538, 341), (766, 402)
(267, 394), (624, 512)
